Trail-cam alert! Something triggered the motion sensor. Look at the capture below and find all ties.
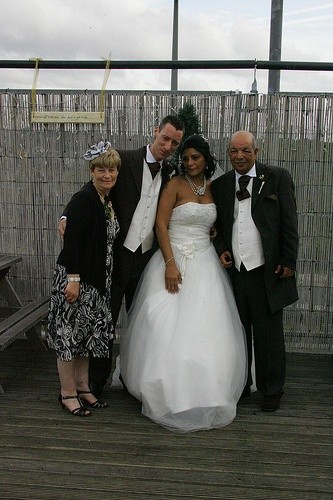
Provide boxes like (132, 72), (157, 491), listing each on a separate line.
(147, 161), (161, 180)
(235, 174), (252, 201)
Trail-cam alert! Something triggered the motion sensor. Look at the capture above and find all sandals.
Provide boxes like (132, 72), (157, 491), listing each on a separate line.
(58, 392), (92, 417)
(75, 388), (111, 409)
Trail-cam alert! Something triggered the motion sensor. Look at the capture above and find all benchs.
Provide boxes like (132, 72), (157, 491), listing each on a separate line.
(0, 296), (50, 393)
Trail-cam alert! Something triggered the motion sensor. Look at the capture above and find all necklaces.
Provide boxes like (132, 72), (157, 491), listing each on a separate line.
(185, 174), (207, 196)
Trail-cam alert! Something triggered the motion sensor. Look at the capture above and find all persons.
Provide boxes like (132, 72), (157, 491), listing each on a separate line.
(58, 116), (186, 395)
(210, 130), (299, 412)
(43, 140), (121, 417)
(118, 133), (248, 434)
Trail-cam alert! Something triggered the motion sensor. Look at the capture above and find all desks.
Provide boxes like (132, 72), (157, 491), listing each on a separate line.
(0, 255), (24, 310)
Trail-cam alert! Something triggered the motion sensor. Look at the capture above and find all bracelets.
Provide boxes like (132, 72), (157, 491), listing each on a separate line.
(67, 278), (80, 282)
(165, 256), (175, 265)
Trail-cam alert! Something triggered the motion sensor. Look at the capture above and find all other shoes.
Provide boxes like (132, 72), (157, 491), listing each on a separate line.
(260, 390), (281, 413)
